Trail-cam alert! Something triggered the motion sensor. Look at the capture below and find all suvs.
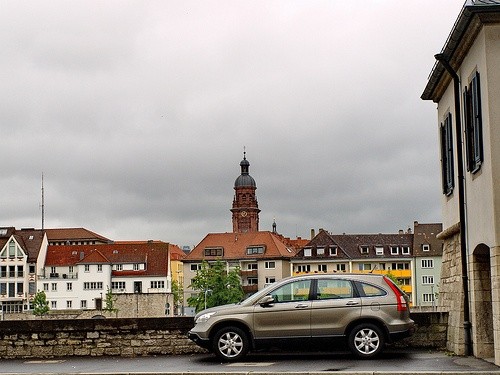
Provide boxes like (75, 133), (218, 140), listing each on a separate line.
(186, 274), (415, 363)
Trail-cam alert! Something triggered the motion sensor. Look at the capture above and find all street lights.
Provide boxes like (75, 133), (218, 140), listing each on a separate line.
(135, 290), (139, 317)
(204, 289), (214, 309)
